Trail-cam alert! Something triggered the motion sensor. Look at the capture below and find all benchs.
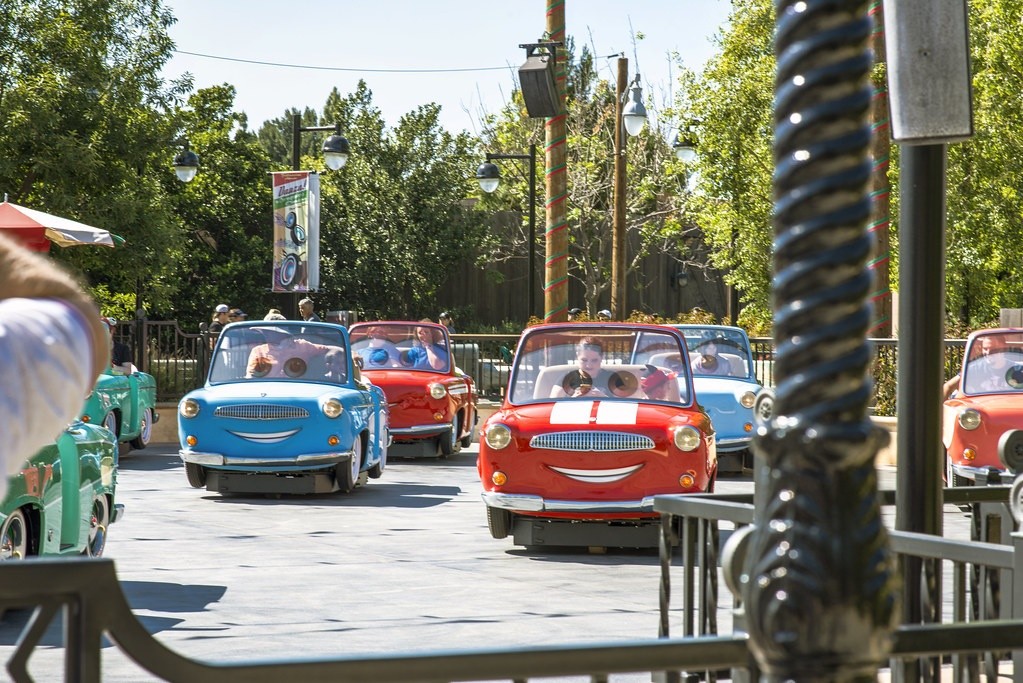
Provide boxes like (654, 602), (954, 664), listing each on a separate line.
(397, 347), (456, 373)
(648, 352), (746, 378)
(302, 346), (361, 381)
(533, 364), (681, 403)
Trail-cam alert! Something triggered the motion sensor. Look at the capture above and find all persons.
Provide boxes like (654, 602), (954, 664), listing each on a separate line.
(102, 316), (131, 374)
(640, 365), (678, 399)
(246, 308), (346, 378)
(568, 308), (581, 321)
(944, 334), (1023, 400)
(598, 310), (611, 320)
(690, 342), (732, 375)
(0, 231), (105, 498)
(405, 318), (447, 371)
(551, 337), (625, 396)
(439, 312), (455, 334)
(299, 298), (320, 334)
(208, 305), (248, 368)
(354, 326), (402, 367)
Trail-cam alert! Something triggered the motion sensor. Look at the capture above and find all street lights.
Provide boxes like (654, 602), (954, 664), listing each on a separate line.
(610, 52), (647, 322)
(476, 145), (537, 327)
(136, 135), (199, 372)
(287, 114), (349, 319)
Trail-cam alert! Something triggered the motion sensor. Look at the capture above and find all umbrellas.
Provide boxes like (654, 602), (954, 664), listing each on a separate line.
(0, 194), (126, 251)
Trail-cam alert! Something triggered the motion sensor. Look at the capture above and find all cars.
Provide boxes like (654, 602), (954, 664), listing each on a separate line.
(476, 321), (717, 556)
(0, 415), (124, 565)
(337, 322), (479, 456)
(62, 362), (159, 458)
(178, 319), (393, 497)
(941, 326), (1023, 517)
(625, 324), (769, 475)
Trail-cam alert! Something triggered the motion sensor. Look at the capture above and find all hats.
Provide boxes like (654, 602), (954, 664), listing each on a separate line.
(216, 304), (229, 312)
(439, 312), (450, 319)
(234, 310), (247, 318)
(639, 365), (679, 393)
(567, 308), (582, 314)
(248, 313), (294, 337)
(598, 309), (611, 320)
